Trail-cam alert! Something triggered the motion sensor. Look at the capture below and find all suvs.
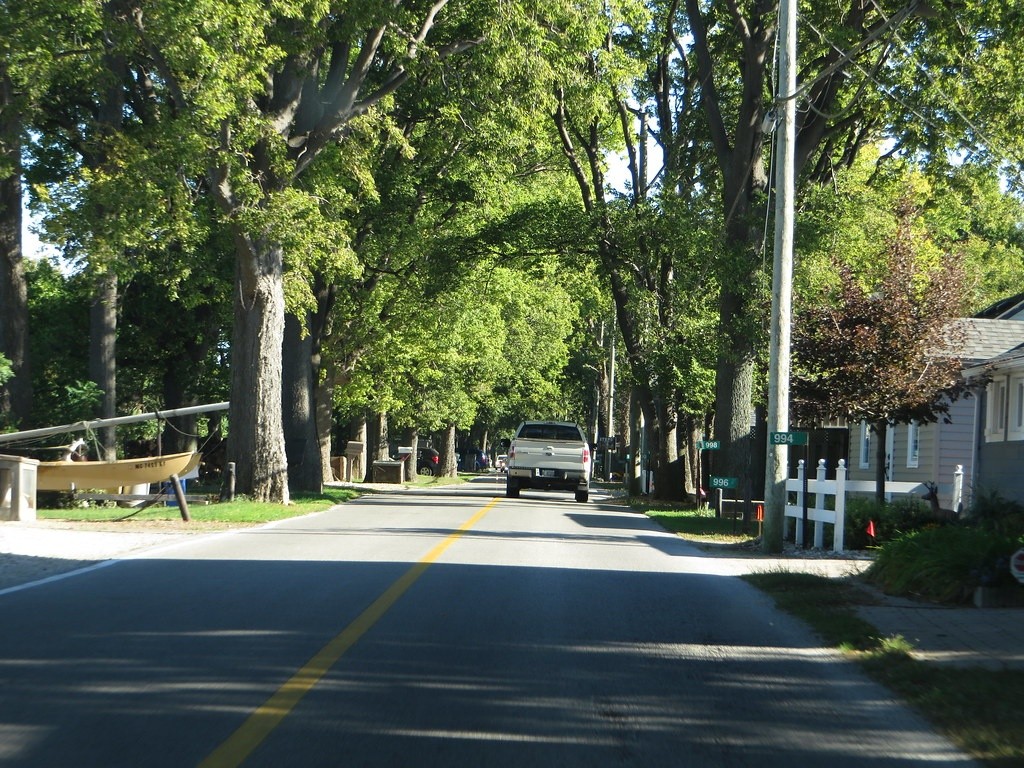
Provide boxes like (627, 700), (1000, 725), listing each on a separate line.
(455, 447), (488, 472)
(500, 420), (598, 503)
(390, 447), (440, 477)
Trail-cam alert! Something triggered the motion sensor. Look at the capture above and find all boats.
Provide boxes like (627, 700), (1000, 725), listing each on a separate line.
(34, 451), (203, 491)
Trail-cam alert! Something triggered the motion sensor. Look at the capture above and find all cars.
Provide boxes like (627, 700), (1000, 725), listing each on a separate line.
(495, 454), (508, 470)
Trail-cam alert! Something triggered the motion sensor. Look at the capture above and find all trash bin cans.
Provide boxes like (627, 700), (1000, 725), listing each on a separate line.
(164, 479), (187, 506)
